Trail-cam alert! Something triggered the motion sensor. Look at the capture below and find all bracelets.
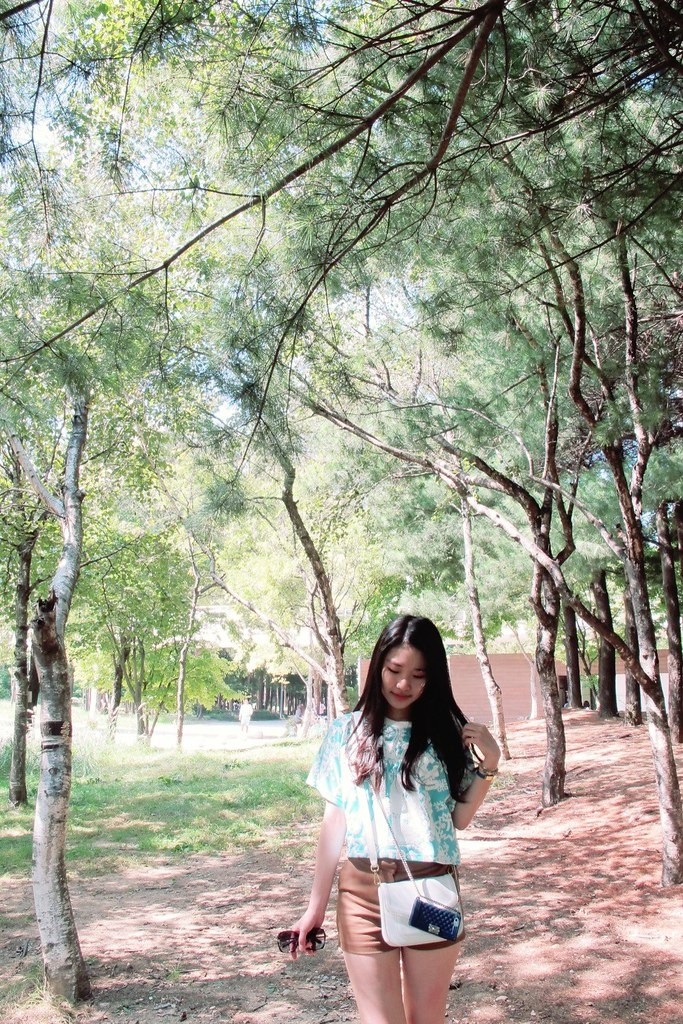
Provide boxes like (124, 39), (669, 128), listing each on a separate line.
(478, 763), (498, 775)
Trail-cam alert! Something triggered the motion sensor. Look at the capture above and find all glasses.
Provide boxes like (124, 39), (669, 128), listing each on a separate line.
(276, 927), (326, 953)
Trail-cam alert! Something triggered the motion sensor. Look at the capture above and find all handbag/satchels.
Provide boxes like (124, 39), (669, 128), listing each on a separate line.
(372, 870), (465, 947)
(407, 896), (460, 942)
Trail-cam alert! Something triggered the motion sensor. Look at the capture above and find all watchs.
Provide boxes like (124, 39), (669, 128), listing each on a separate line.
(476, 770), (494, 780)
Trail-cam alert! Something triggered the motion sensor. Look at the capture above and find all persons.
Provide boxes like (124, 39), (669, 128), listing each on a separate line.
(239, 698), (253, 735)
(271, 614), (501, 1024)
